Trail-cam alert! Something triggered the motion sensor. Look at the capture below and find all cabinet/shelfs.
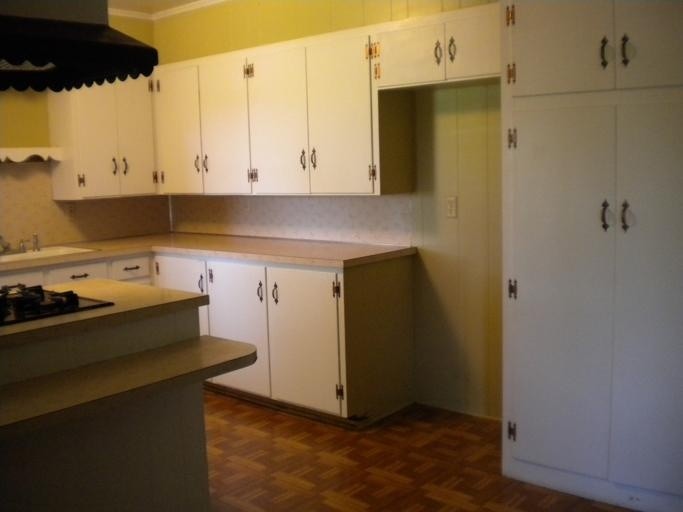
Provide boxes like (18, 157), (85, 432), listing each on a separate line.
(155, 26), (380, 197)
(151, 253), (210, 385)
(503, 0), (682, 511)
(45, 64), (87, 202)
(0, 251), (150, 286)
(90, 71), (151, 200)
(213, 257), (416, 430)
(377, 2), (502, 91)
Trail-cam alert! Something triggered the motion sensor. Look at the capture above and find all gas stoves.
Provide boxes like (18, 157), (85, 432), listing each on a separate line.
(0, 282), (114, 326)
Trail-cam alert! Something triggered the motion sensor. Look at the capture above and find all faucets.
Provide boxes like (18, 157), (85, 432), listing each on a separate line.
(30, 233), (41, 252)
(0, 236), (11, 251)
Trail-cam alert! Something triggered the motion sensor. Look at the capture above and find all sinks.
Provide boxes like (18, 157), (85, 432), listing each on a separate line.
(0, 246), (102, 262)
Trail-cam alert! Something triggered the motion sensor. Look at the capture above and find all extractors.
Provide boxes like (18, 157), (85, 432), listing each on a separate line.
(0, 0), (158, 92)
